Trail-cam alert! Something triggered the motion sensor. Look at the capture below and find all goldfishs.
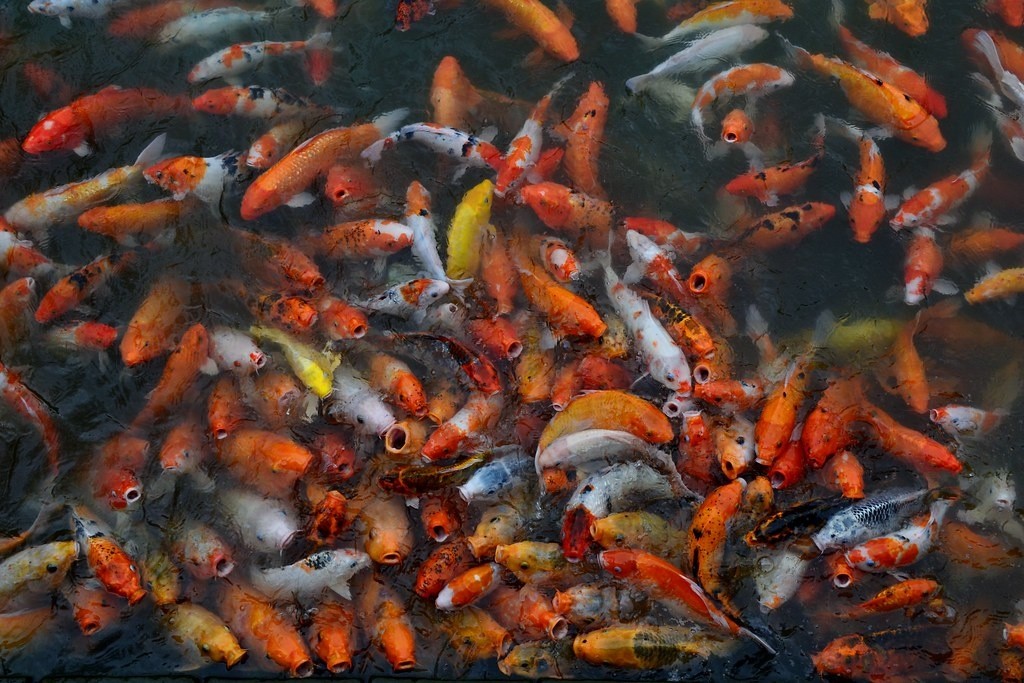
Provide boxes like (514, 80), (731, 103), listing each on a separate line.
(0, 0), (1024, 683)
